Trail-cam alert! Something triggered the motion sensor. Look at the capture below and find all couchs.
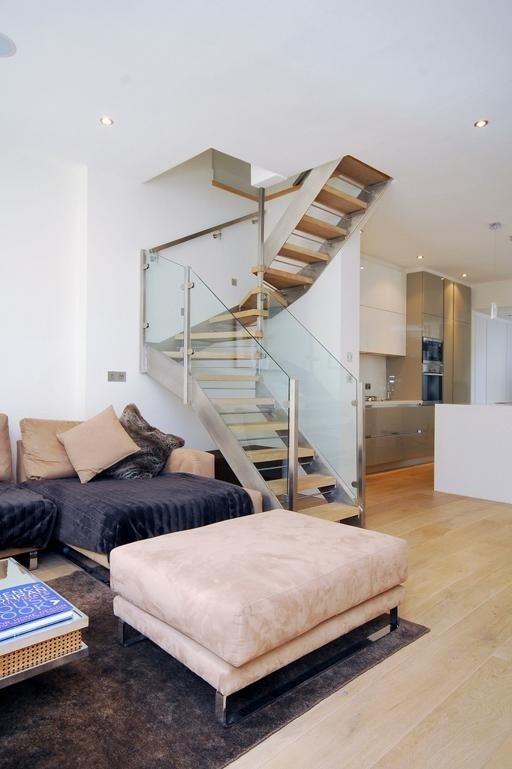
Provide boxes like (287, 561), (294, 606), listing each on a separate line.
(1, 413), (264, 571)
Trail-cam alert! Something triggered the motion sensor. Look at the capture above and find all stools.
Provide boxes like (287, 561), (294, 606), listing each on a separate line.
(107, 508), (410, 726)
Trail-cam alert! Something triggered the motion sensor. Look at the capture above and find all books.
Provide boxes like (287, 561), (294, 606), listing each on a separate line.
(1, 581), (74, 643)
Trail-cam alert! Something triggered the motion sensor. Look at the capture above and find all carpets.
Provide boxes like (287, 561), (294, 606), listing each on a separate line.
(0, 569), (429, 768)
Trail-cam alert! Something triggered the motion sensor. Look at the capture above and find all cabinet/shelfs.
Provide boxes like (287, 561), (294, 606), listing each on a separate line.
(360, 253), (512, 504)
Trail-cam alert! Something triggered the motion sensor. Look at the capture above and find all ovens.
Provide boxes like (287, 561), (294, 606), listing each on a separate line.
(420, 336), (444, 403)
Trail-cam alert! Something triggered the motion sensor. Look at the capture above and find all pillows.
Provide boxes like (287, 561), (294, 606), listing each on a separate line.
(56, 404), (142, 485)
(107, 404), (187, 482)
